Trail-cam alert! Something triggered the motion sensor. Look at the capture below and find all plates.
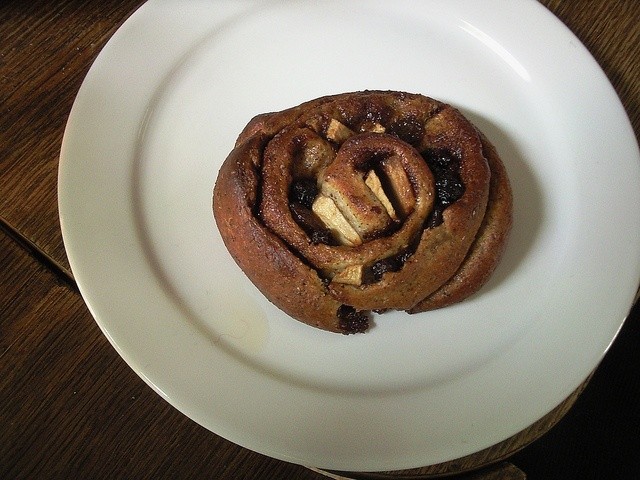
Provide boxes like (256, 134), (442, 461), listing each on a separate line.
(59, 1), (639, 472)
